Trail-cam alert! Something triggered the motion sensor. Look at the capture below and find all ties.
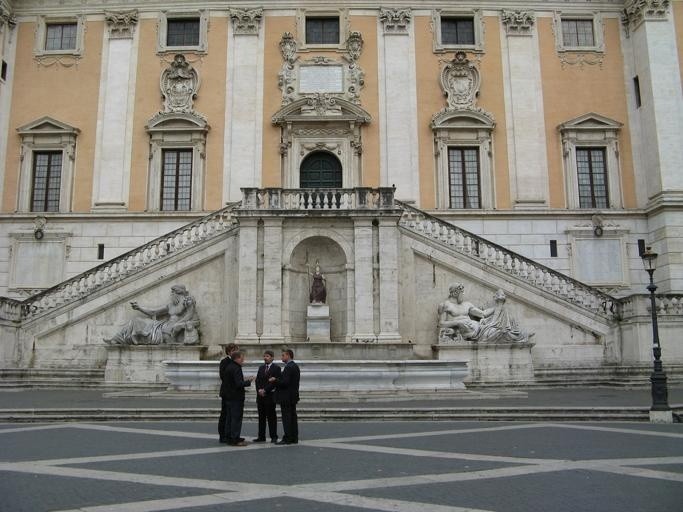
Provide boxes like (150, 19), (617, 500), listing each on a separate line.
(265, 366), (269, 375)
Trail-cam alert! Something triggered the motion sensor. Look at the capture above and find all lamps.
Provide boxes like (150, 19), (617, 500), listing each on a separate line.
(257, 217), (265, 226)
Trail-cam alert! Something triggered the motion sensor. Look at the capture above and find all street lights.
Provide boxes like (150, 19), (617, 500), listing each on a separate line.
(642, 247), (670, 410)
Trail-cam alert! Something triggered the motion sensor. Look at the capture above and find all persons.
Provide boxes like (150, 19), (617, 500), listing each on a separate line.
(101, 282), (202, 345)
(252, 351), (281, 443)
(306, 259), (327, 304)
(217, 343), (237, 444)
(221, 351), (255, 446)
(268, 349), (299, 444)
(437, 281), (536, 343)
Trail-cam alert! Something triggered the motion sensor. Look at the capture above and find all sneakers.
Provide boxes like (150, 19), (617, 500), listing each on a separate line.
(219, 437), (299, 447)
